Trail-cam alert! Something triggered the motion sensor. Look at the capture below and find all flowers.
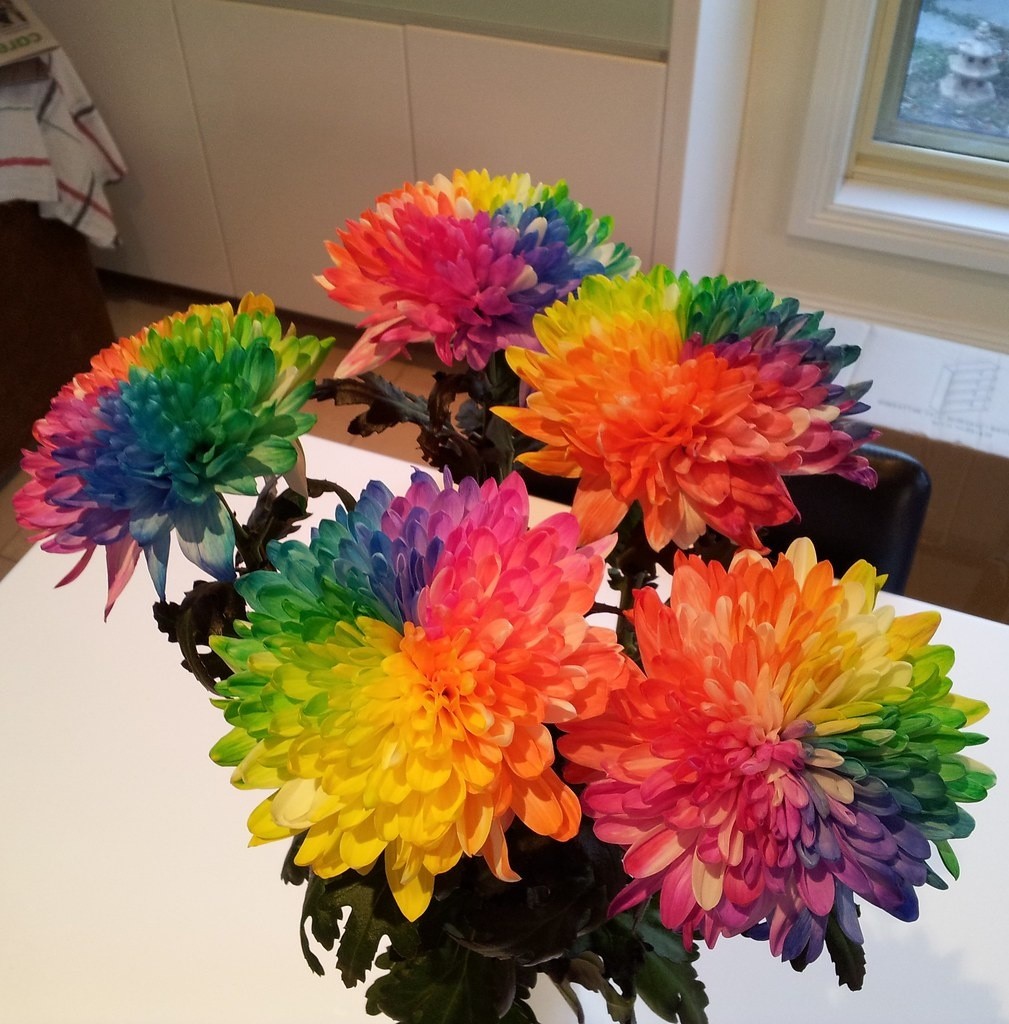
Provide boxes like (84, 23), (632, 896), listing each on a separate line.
(9, 163), (1002, 1024)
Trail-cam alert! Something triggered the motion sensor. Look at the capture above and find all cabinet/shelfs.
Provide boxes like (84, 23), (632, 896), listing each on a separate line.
(0, 0), (762, 353)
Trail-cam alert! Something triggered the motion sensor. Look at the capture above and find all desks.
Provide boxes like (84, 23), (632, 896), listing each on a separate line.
(0, 444), (1009, 1024)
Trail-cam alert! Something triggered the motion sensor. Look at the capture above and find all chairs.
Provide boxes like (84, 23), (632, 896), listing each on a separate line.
(761, 431), (933, 596)
